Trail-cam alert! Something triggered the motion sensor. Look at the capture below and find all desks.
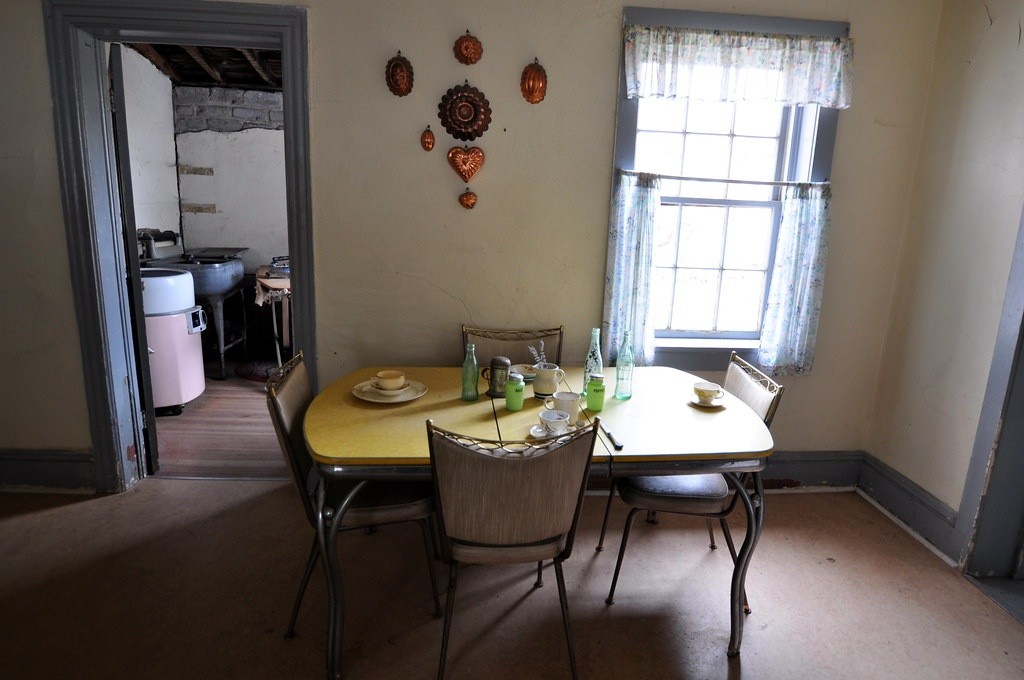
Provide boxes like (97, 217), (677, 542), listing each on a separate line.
(256, 265), (291, 346)
(304, 364), (773, 649)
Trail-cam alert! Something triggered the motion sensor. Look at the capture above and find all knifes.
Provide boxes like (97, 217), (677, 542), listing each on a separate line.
(600, 421), (624, 449)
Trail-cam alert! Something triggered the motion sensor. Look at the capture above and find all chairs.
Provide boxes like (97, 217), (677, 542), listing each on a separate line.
(459, 323), (563, 364)
(422, 416), (600, 680)
(266, 355), (441, 641)
(596, 349), (785, 610)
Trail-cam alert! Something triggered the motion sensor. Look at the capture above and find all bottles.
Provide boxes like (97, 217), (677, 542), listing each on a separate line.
(506, 373), (526, 411)
(582, 327), (603, 393)
(462, 343), (478, 401)
(614, 333), (635, 400)
(587, 375), (604, 412)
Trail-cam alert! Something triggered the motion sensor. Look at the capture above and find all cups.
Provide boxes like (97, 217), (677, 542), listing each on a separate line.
(481, 356), (512, 398)
(538, 410), (571, 438)
(693, 381), (724, 404)
(533, 363), (565, 401)
(371, 371), (405, 390)
(544, 391), (581, 426)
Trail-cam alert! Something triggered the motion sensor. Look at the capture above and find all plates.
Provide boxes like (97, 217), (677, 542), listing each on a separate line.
(352, 377), (430, 404)
(508, 363), (538, 381)
(370, 380), (411, 396)
(691, 395), (725, 407)
(529, 424), (580, 440)
